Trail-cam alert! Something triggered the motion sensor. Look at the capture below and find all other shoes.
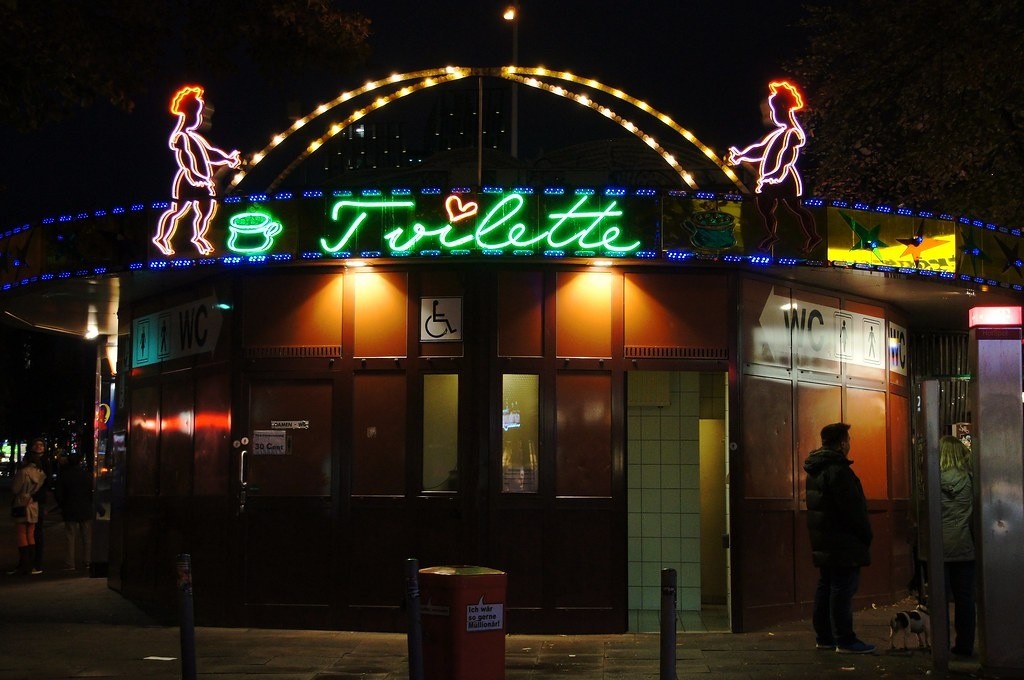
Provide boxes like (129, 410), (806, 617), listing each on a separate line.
(85, 560), (90, 568)
(951, 646), (972, 658)
(32, 567), (43, 574)
(64, 565), (75, 570)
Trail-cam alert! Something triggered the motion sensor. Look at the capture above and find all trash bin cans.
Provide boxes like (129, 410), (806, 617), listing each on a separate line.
(419, 565), (508, 680)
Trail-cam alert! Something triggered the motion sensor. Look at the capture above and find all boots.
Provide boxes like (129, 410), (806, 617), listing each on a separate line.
(7, 544), (35, 576)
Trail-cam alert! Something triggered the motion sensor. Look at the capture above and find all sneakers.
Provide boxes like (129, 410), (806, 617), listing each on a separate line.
(815, 636), (835, 648)
(836, 639), (877, 653)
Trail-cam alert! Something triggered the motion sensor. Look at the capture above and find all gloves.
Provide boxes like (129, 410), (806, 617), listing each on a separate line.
(100, 509), (106, 517)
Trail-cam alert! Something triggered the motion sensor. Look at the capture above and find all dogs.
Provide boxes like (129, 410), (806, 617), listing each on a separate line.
(880, 596), (930, 650)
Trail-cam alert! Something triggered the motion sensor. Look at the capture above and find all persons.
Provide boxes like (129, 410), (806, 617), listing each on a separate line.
(30, 438), (51, 574)
(918, 434), (977, 654)
(803, 423), (875, 652)
(12, 450), (46, 575)
(57, 454), (93, 571)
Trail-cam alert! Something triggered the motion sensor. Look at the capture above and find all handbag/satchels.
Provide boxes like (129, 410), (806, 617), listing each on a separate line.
(12, 506), (26, 518)
